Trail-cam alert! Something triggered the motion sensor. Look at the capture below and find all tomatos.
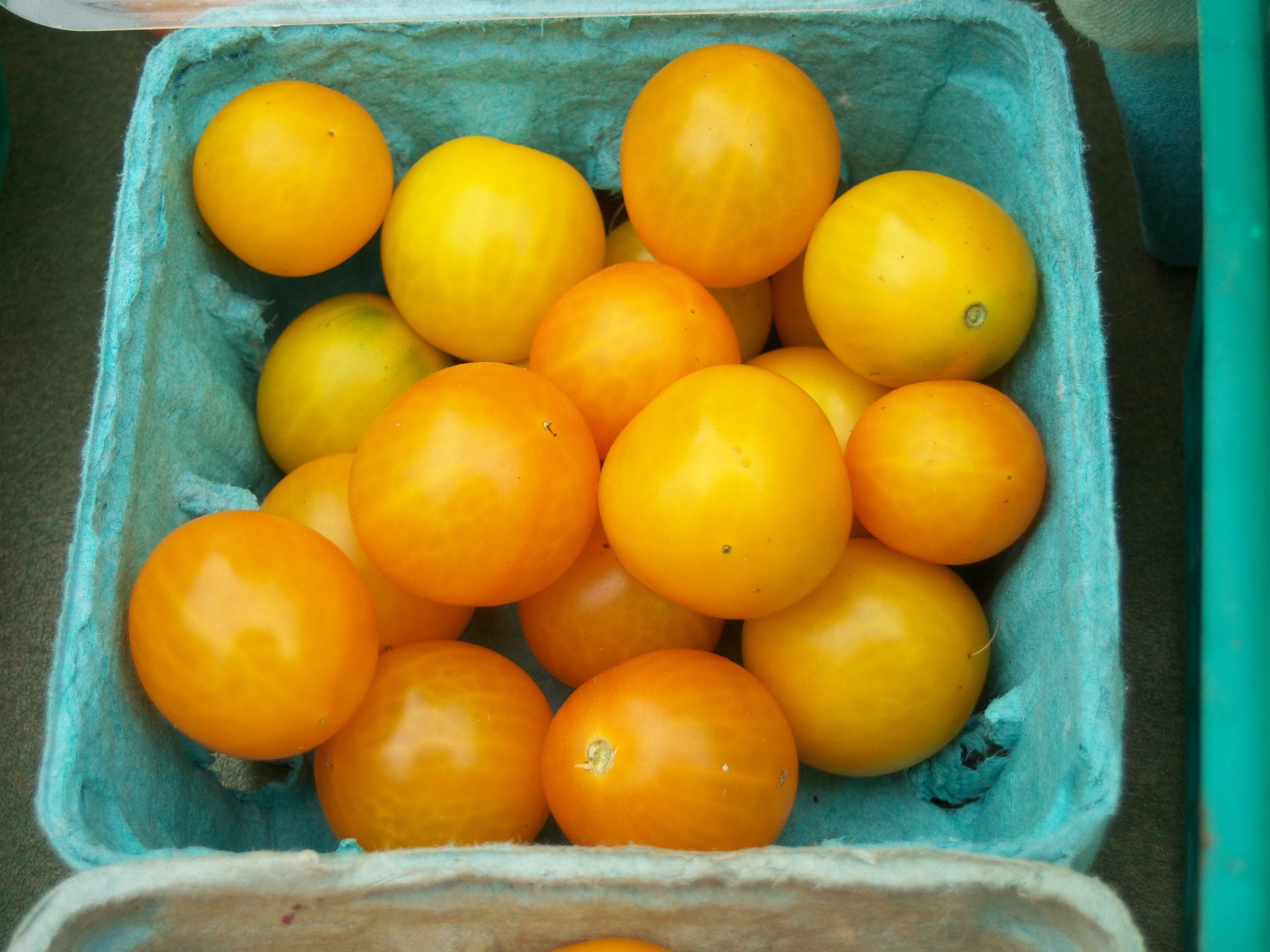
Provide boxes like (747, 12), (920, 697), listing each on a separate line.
(547, 936), (670, 952)
(124, 44), (1048, 858)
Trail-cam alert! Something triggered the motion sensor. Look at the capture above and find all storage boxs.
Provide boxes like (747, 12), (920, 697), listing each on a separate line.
(0, 0), (1269, 952)
